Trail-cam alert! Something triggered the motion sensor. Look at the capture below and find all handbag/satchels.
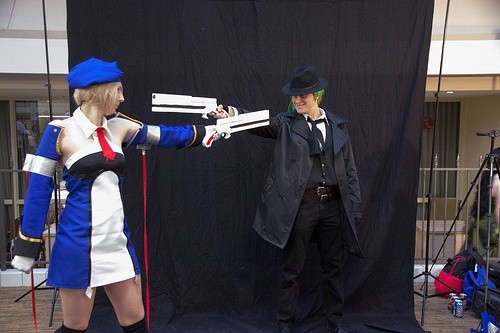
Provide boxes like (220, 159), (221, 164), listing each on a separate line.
(433, 247), (500, 304)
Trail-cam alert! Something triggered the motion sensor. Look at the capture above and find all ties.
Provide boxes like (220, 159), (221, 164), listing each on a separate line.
(96, 127), (116, 161)
(307, 117), (326, 156)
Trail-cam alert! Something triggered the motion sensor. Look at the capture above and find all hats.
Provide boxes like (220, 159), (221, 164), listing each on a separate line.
(281, 66), (328, 95)
(66, 57), (124, 89)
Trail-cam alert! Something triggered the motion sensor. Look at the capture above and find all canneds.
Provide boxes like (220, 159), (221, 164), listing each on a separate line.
(448, 293), (468, 318)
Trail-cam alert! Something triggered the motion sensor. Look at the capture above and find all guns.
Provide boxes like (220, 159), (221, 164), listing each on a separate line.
(151, 92), (231, 123)
(201, 109), (270, 148)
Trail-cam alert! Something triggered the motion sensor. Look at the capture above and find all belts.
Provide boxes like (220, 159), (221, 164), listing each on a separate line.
(303, 186), (338, 202)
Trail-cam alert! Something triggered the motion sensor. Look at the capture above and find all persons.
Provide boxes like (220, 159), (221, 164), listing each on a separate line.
(11, 56), (224, 333)
(209, 65), (366, 333)
(462, 148), (500, 259)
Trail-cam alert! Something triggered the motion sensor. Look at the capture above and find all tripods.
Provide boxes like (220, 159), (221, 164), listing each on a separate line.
(419, 130), (500, 333)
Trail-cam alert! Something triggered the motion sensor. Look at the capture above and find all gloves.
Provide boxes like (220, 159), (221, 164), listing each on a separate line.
(204, 125), (221, 142)
(11, 255), (35, 274)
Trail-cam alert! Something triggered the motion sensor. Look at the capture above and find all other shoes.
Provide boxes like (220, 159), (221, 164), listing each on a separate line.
(326, 321), (351, 333)
(278, 325), (292, 333)
